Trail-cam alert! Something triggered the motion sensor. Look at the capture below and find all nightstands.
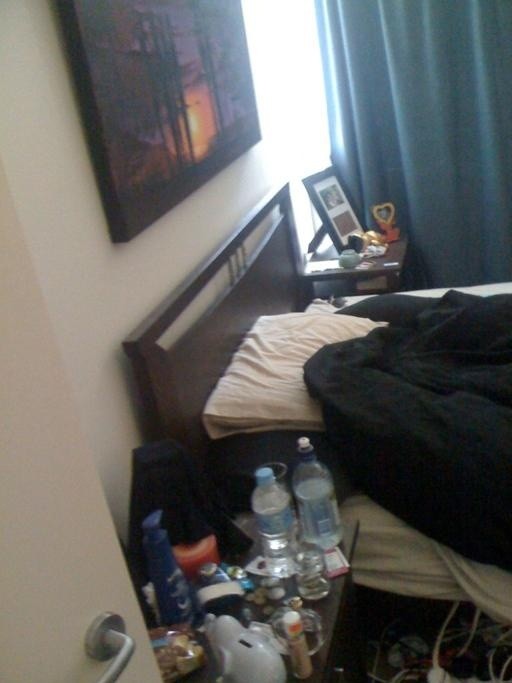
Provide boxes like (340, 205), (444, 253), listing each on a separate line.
(303, 230), (418, 300)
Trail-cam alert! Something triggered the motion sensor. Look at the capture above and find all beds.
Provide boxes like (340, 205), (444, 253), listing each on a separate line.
(121, 180), (511, 621)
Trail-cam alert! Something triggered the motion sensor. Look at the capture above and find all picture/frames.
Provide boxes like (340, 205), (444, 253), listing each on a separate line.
(301, 164), (368, 255)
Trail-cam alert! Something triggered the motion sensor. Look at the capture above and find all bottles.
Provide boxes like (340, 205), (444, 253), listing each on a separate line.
(250, 466), (293, 579)
(291, 438), (343, 547)
(338, 248), (359, 270)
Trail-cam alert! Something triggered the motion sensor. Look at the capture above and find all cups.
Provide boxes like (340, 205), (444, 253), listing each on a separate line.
(272, 609), (324, 679)
(255, 460), (287, 492)
(294, 546), (333, 602)
